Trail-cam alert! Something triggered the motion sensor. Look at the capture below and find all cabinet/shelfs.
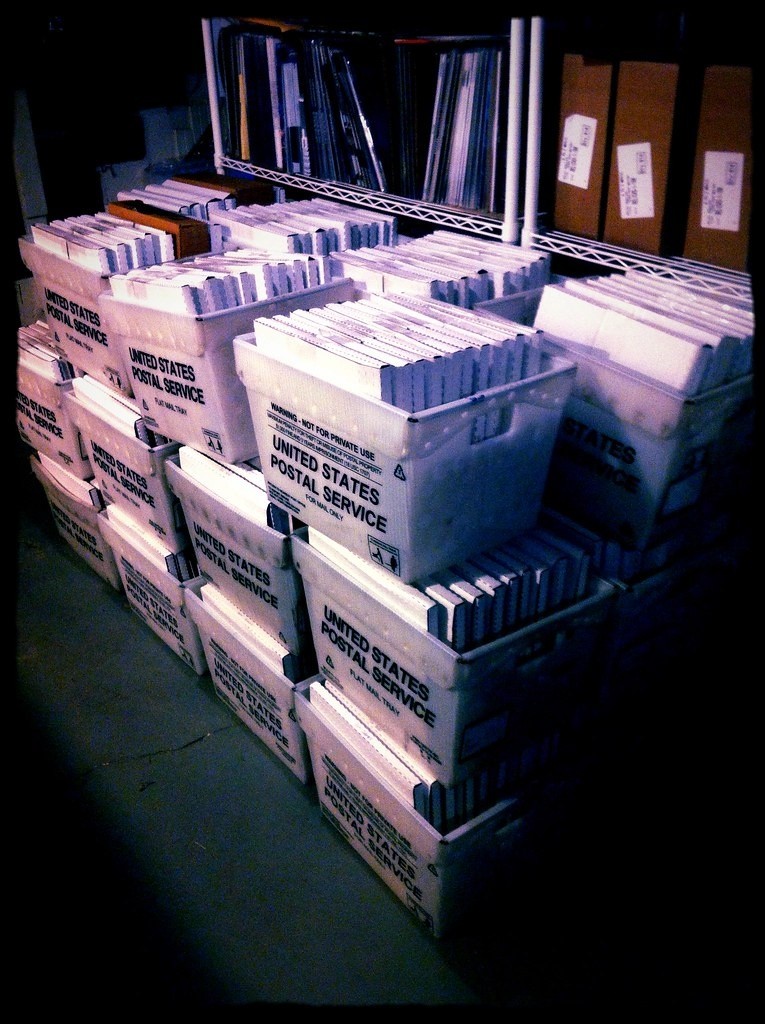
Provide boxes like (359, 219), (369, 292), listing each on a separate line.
(200, 14), (525, 259)
(527, 6), (762, 306)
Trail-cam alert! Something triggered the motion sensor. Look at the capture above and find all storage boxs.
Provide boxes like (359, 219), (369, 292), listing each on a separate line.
(556, 43), (755, 270)
(15, 178), (756, 940)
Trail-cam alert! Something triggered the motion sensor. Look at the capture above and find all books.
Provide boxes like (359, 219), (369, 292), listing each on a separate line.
(206, 8), (508, 216)
